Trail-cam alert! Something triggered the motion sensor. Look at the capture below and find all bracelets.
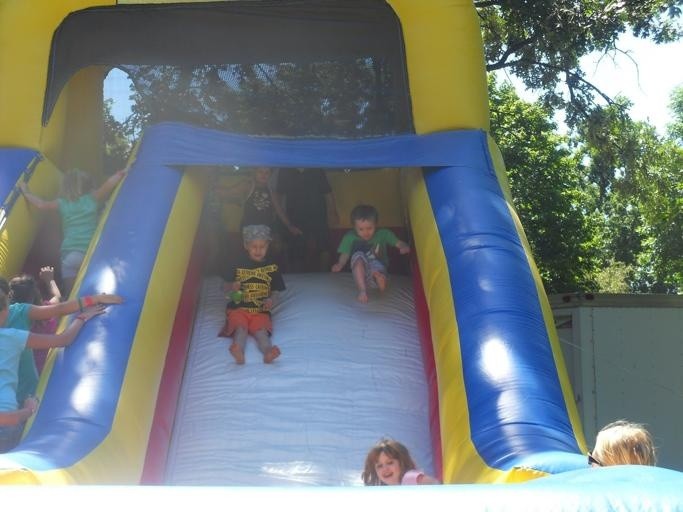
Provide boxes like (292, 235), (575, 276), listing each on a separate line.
(118, 170), (125, 177)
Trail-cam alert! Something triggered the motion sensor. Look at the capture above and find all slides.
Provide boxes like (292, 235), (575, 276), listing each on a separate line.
(0, 121), (592, 487)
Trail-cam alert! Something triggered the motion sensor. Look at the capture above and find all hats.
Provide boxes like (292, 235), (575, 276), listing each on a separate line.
(243, 224), (273, 243)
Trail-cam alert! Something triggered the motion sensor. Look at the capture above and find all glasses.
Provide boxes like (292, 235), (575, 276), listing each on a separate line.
(587, 451), (601, 465)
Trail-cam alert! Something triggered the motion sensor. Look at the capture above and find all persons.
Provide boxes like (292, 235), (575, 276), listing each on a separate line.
(331, 206), (410, 303)
(0, 265), (124, 455)
(17, 166), (130, 296)
(269, 167), (339, 272)
(361, 438), (442, 484)
(209, 166), (304, 238)
(587, 419), (656, 466)
(218, 224), (286, 364)
